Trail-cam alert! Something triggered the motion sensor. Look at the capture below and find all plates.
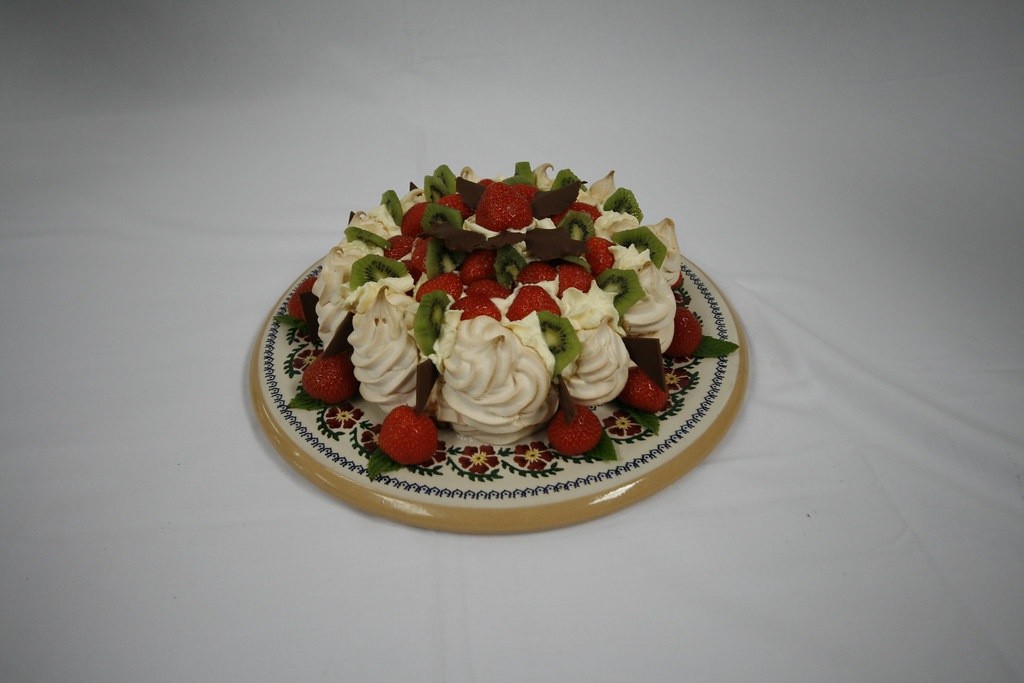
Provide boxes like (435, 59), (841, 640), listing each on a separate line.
(249, 249), (747, 534)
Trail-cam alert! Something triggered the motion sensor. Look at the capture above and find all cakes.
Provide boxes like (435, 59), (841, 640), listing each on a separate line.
(272, 160), (741, 482)
